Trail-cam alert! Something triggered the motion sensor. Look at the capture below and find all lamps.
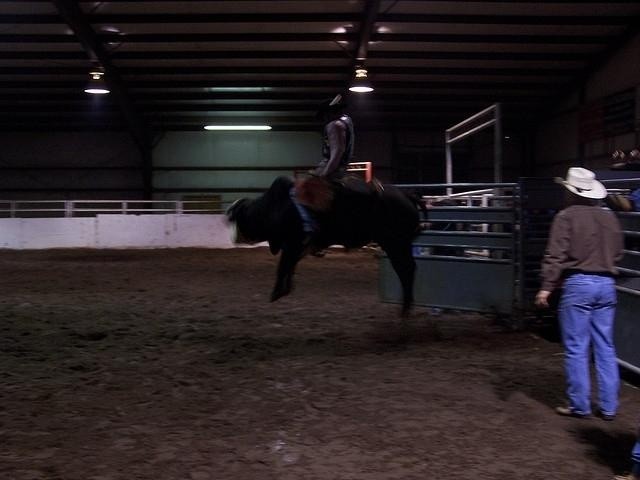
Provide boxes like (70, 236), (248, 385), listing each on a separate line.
(82, 65), (111, 95)
(349, 62), (377, 92)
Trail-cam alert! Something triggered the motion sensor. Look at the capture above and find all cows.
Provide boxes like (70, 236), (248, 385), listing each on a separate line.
(223, 174), (428, 318)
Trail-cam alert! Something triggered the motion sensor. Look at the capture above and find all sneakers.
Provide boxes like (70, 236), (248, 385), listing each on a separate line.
(594, 406), (617, 420)
(302, 234), (313, 245)
(556, 405), (593, 418)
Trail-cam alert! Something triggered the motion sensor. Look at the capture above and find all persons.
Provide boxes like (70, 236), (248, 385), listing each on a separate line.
(534, 165), (621, 420)
(307, 98), (356, 176)
(613, 440), (640, 480)
(407, 162), (640, 326)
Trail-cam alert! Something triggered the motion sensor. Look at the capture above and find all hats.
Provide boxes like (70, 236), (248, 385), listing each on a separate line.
(314, 93), (349, 121)
(553, 167), (608, 200)
